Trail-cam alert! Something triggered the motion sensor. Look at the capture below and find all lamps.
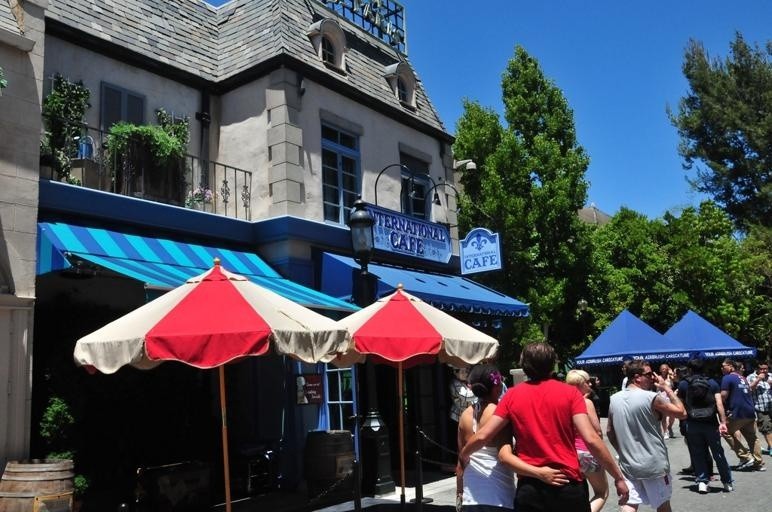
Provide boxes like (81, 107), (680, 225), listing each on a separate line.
(400, 172), (442, 215)
(375, 164), (416, 206)
(424, 183), (463, 222)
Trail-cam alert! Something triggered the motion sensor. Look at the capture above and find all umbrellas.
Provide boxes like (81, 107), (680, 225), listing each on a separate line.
(661, 310), (757, 361)
(73, 256), (355, 512)
(327, 282), (500, 512)
(575, 306), (695, 366)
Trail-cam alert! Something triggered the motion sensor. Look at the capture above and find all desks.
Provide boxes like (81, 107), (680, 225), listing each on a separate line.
(67, 157), (113, 194)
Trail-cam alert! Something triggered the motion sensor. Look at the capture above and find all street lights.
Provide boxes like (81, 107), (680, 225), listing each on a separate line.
(347, 191), (397, 494)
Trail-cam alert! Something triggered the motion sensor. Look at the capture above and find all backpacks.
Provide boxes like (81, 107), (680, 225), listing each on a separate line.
(683, 376), (716, 418)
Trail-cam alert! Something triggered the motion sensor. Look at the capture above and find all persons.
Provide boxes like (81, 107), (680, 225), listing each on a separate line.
(458, 341), (630, 512)
(450, 355), (772, 512)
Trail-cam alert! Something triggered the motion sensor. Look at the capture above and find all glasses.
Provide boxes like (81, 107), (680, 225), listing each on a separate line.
(640, 372), (653, 379)
(720, 364), (730, 369)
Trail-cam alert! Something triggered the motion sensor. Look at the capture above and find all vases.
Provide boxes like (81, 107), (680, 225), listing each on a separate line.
(193, 202), (213, 213)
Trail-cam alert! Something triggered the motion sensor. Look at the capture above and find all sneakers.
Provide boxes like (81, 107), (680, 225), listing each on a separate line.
(725, 483), (736, 492)
(699, 481), (712, 493)
(683, 466), (694, 473)
(668, 426), (673, 438)
(736, 457), (755, 470)
(750, 464), (767, 471)
(664, 432), (669, 440)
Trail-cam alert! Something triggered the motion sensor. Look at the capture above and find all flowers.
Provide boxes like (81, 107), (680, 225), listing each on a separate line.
(188, 187), (218, 206)
(488, 371), (505, 386)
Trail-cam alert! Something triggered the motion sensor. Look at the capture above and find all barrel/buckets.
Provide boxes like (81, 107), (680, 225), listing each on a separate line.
(0, 456), (76, 512)
(306, 428), (357, 503)
